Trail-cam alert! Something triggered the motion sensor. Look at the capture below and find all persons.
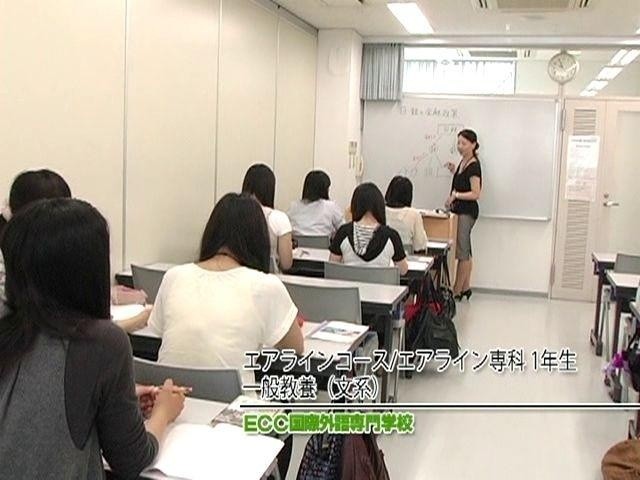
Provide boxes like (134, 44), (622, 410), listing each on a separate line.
(0, 199), (184, 480)
(285, 171), (345, 242)
(328, 182), (408, 276)
(242, 163), (293, 275)
(385, 176), (429, 256)
(0, 169), (152, 334)
(146, 192), (303, 480)
(444, 129), (482, 302)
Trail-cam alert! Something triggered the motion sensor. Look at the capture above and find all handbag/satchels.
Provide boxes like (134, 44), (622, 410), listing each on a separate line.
(405, 286), (458, 359)
(296, 412), (389, 480)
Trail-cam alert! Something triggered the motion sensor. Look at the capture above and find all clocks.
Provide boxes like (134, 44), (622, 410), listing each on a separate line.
(546, 51), (580, 85)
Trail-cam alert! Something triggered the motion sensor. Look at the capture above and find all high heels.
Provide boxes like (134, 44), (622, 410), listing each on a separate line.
(452, 289), (472, 302)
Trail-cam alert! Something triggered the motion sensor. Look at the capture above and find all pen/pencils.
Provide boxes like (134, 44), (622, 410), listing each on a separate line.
(158, 386), (192, 392)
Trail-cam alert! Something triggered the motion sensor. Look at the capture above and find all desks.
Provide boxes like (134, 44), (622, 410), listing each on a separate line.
(586, 252), (640, 439)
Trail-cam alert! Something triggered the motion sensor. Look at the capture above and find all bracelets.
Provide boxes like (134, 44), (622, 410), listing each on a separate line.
(452, 190), (457, 200)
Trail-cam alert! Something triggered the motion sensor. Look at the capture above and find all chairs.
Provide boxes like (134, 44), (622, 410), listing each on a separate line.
(98, 234), (456, 479)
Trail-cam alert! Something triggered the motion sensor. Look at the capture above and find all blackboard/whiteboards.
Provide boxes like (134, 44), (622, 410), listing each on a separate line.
(357, 94), (565, 222)
